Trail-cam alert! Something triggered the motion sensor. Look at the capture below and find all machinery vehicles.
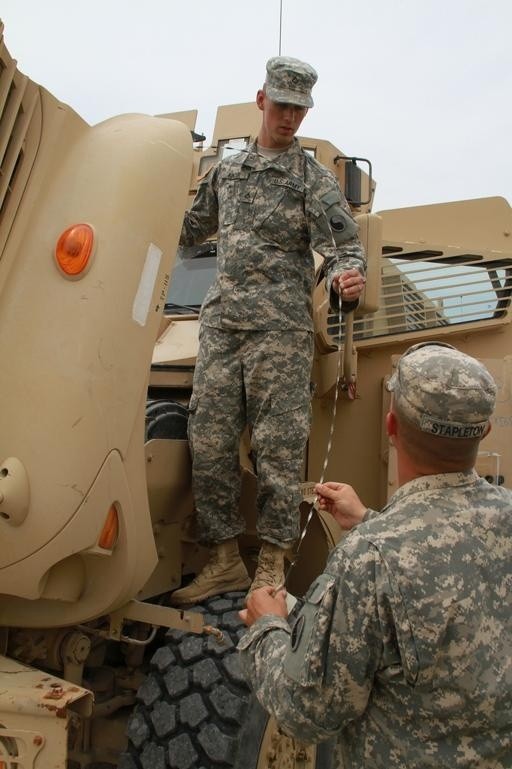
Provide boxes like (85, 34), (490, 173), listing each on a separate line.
(0, 19), (512, 768)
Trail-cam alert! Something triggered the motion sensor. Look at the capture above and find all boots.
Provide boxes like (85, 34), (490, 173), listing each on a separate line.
(244, 539), (286, 606)
(169, 536), (252, 607)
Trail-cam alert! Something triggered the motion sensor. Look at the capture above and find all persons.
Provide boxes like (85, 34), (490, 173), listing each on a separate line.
(164, 54), (371, 624)
(232, 340), (511, 768)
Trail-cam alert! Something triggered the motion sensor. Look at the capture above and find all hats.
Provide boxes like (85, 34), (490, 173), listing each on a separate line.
(385, 341), (499, 441)
(264, 55), (318, 108)
